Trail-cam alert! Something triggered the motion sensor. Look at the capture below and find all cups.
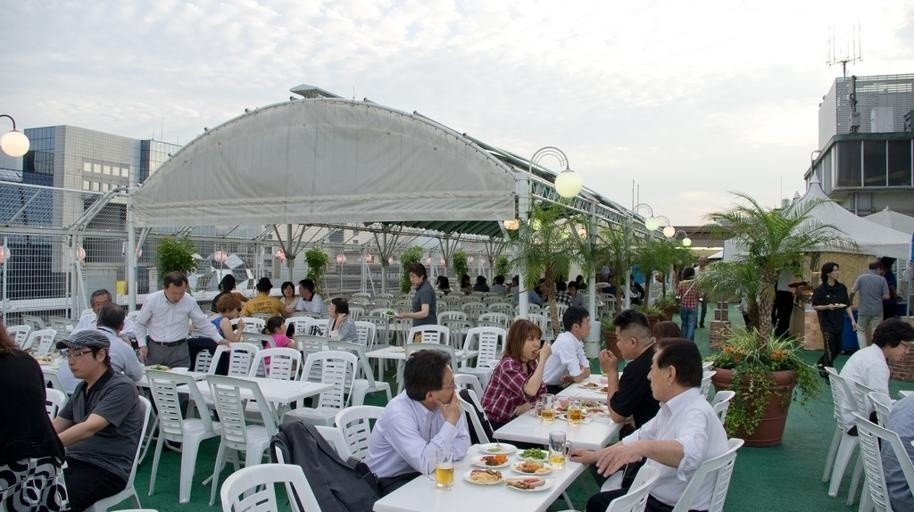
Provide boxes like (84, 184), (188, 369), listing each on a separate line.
(549, 431), (572, 471)
(534, 394), (606, 424)
(435, 451), (454, 490)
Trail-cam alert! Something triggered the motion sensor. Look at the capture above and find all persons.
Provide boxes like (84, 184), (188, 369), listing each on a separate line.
(676, 267), (698, 342)
(188, 272), (361, 409)
(540, 304), (590, 394)
(49, 329), (143, 511)
(768, 254), (913, 511)
(392, 262), (438, 334)
(567, 338), (730, 512)
(693, 255), (711, 328)
(433, 272), (645, 309)
(361, 347), (472, 496)
(0, 313), (72, 511)
(55, 301), (143, 400)
(596, 310), (660, 440)
(132, 271), (231, 452)
(479, 318), (552, 449)
(650, 320), (681, 343)
(58, 288), (134, 354)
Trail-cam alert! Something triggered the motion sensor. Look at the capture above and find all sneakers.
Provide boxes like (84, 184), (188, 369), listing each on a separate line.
(818, 359), (835, 385)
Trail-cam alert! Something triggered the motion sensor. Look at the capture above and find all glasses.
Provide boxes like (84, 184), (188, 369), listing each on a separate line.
(64, 350), (94, 358)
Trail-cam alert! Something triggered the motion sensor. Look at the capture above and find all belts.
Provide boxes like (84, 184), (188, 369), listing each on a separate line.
(149, 336), (186, 346)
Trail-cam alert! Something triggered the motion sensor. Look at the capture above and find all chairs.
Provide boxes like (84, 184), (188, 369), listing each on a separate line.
(858, 391), (892, 512)
(848, 382), (874, 506)
(852, 412), (914, 512)
(823, 367), (859, 496)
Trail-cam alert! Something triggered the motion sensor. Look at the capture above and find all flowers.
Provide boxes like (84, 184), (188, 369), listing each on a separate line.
(704, 323), (822, 439)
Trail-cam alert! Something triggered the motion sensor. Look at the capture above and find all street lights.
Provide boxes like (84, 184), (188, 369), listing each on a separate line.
(672, 229), (691, 248)
(648, 214), (675, 286)
(622, 201), (659, 312)
(511, 146), (580, 335)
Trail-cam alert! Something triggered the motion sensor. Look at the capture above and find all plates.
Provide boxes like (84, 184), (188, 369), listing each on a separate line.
(35, 351), (61, 370)
(576, 380), (607, 392)
(463, 442), (553, 492)
(382, 311), (397, 317)
(384, 347), (404, 353)
(832, 303), (847, 309)
(144, 364), (167, 371)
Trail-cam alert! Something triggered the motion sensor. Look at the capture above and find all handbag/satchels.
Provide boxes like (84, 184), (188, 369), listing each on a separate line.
(675, 293), (681, 304)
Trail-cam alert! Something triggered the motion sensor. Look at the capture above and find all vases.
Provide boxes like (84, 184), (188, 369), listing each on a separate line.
(712, 368), (798, 447)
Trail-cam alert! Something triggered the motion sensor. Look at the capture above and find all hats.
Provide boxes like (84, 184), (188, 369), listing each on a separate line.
(257, 277), (273, 291)
(56, 330), (110, 350)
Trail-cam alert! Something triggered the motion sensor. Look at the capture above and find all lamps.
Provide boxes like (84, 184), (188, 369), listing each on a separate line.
(633, 203), (658, 230)
(656, 215), (675, 237)
(675, 230), (691, 246)
(529, 147), (582, 211)
